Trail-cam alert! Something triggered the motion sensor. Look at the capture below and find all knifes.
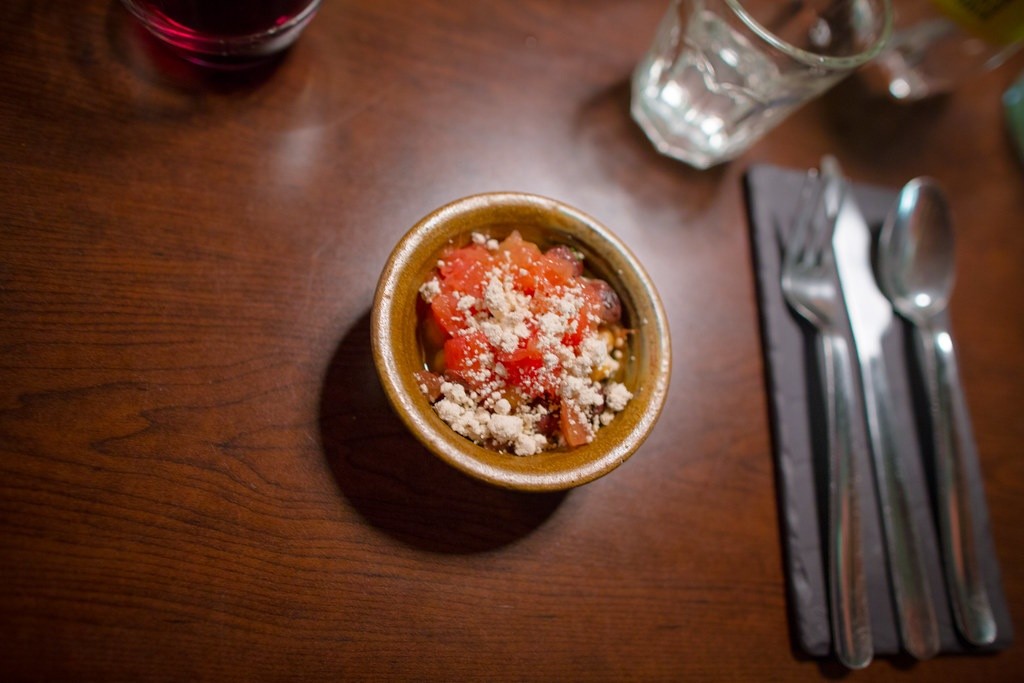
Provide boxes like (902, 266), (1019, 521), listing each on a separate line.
(820, 156), (938, 659)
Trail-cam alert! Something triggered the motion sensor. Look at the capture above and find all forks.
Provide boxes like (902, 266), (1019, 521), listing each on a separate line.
(781, 168), (874, 668)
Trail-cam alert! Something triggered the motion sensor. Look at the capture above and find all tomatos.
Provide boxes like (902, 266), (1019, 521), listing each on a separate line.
(418, 229), (626, 448)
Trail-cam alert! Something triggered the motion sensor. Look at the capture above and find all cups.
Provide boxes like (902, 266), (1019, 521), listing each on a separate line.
(121, 1), (321, 95)
(626, 0), (1024, 171)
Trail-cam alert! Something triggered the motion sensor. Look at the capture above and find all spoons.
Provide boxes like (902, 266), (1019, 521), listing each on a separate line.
(877, 178), (1005, 646)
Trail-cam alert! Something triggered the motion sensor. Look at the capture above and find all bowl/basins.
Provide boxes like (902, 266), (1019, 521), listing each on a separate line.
(370, 192), (672, 492)
